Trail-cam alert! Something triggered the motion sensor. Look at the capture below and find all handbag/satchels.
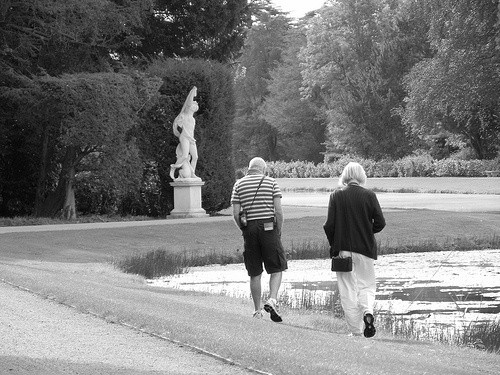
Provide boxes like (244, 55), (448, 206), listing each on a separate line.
(239, 210), (248, 227)
(331, 256), (353, 272)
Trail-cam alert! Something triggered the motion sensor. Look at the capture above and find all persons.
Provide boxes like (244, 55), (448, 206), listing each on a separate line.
(231, 157), (288, 321)
(169, 86), (199, 180)
(323, 162), (386, 337)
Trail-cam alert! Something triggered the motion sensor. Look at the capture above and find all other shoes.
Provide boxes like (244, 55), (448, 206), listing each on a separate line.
(362, 310), (377, 337)
(264, 298), (283, 322)
(252, 310), (263, 319)
